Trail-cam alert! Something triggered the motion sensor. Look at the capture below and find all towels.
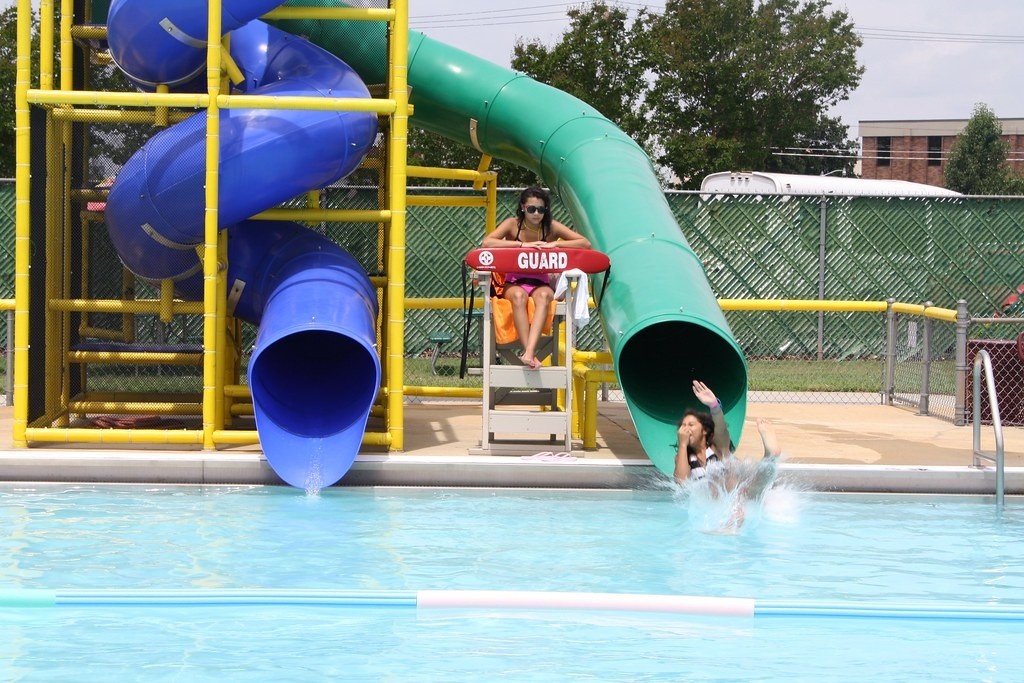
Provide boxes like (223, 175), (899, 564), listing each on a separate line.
(553, 267), (590, 329)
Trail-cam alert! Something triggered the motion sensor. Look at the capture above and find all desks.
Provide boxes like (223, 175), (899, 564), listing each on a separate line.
(459, 308), (484, 367)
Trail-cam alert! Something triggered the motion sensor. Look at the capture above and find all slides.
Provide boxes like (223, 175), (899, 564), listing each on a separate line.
(101, 0), (383, 492)
(263, 0), (749, 484)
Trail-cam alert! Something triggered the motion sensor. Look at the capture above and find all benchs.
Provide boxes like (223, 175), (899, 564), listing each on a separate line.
(429, 332), (452, 376)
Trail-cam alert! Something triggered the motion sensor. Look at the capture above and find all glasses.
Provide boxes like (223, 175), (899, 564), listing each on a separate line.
(523, 205), (548, 214)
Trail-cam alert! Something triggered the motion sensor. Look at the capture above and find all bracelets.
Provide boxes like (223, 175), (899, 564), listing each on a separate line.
(710, 399), (723, 416)
(520, 241), (524, 248)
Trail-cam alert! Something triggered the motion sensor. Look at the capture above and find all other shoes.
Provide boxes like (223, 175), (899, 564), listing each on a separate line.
(518, 354), (542, 370)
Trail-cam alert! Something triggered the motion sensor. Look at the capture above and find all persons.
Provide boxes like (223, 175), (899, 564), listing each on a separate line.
(674, 380), (781, 483)
(482, 187), (591, 372)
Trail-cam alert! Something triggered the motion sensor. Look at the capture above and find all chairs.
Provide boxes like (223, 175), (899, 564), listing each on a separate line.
(471, 268), (580, 452)
